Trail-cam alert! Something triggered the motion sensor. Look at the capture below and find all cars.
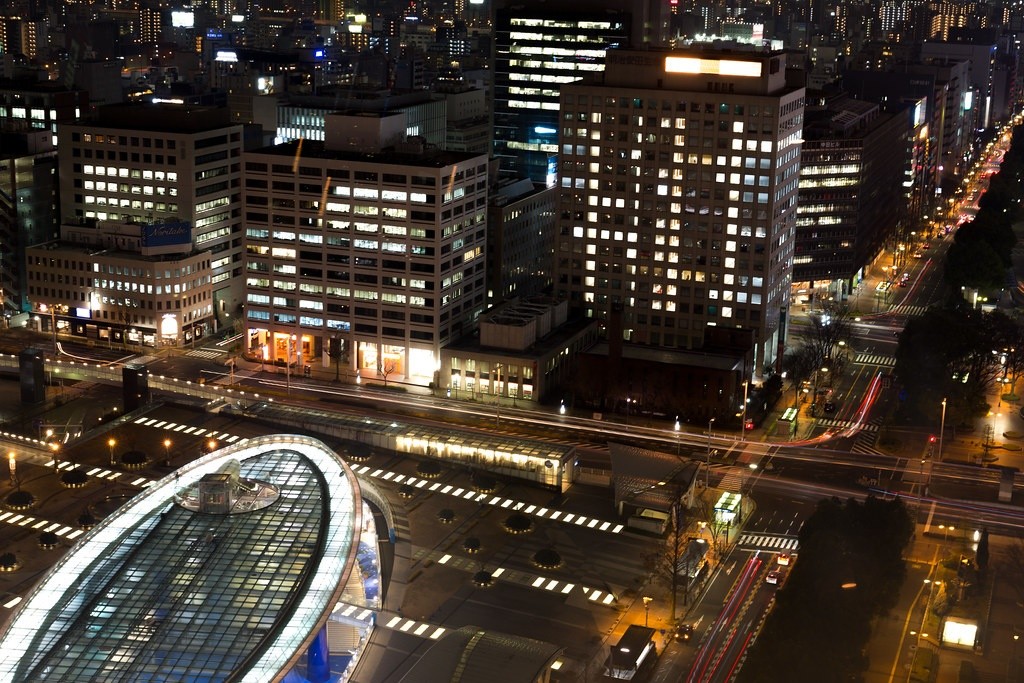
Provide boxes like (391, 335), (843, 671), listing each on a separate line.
(897, 215), (976, 287)
(675, 624), (693, 642)
(766, 571), (780, 585)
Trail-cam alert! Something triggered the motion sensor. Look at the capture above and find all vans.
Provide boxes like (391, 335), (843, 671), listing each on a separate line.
(777, 548), (792, 565)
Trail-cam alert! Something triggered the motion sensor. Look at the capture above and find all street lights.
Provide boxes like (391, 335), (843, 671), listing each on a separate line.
(884, 205), (941, 304)
(846, 318), (861, 359)
(792, 386), (810, 437)
(996, 377), (1010, 411)
(738, 463), (758, 524)
(827, 341), (845, 367)
(286, 332), (298, 395)
(704, 417), (716, 489)
(739, 380), (750, 440)
(810, 367), (829, 417)
